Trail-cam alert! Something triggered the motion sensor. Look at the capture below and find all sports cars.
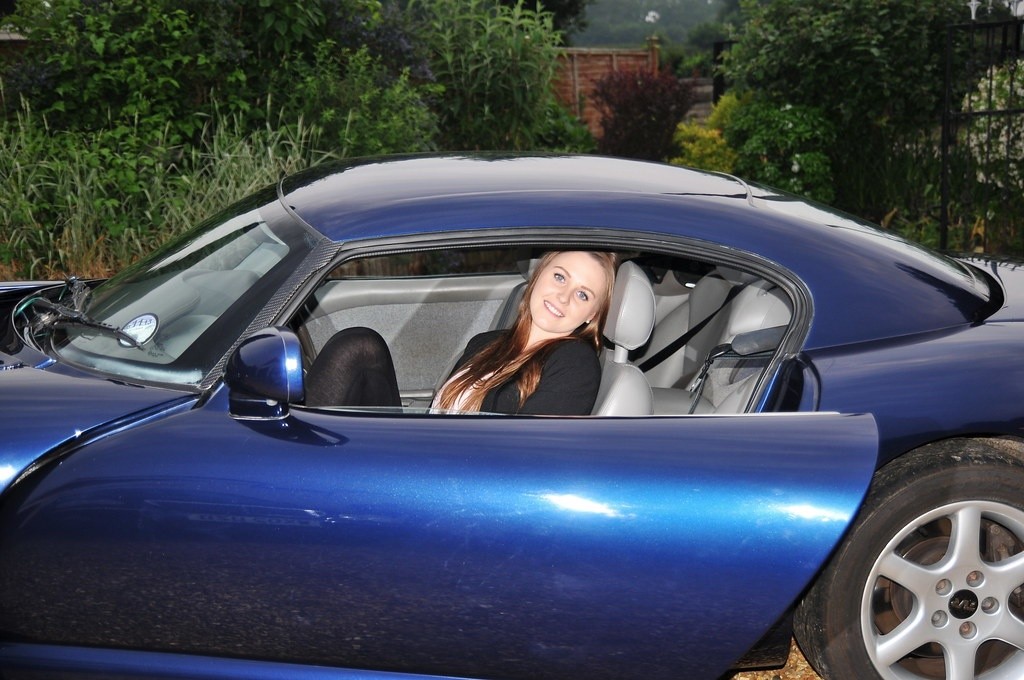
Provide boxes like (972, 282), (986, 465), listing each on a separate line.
(0, 146), (1024, 680)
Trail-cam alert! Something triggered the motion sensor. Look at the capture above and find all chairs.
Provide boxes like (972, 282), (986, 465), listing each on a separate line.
(498, 257), (542, 332)
(586, 262), (656, 417)
(648, 273), (794, 418)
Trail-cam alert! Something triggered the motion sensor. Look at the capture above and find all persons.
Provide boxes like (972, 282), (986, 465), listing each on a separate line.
(303, 250), (614, 427)
